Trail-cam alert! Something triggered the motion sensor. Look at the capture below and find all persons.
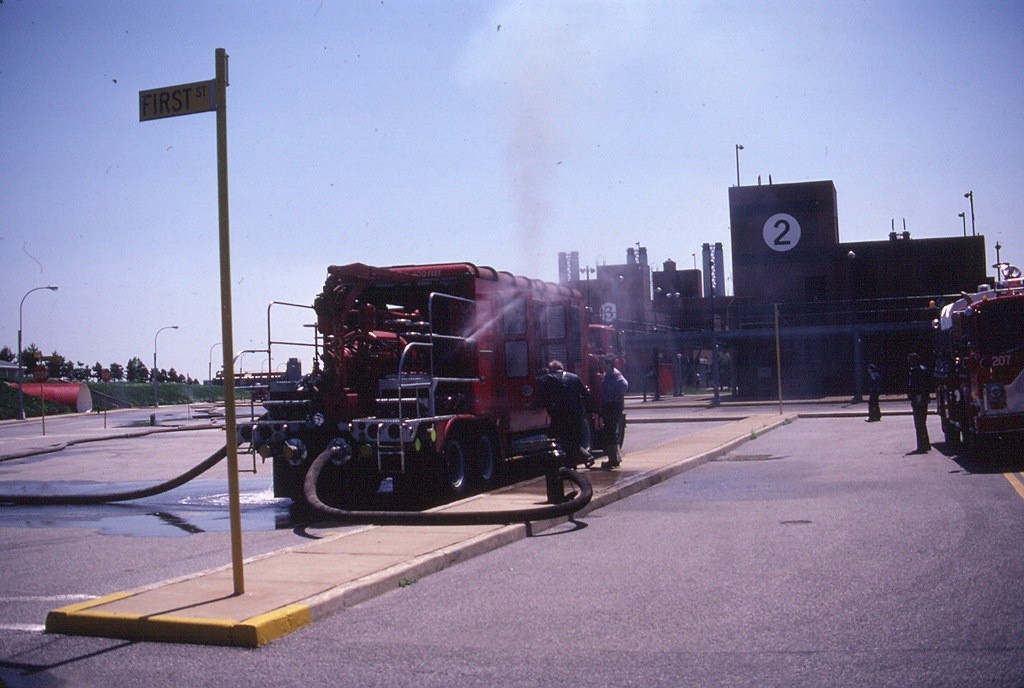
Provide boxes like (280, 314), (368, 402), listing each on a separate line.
(907, 353), (930, 454)
(640, 371), (647, 403)
(601, 357), (629, 469)
(695, 372), (700, 389)
(536, 360), (594, 471)
(867, 363), (881, 422)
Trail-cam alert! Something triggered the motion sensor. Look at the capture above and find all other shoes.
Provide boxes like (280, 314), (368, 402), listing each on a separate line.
(601, 460), (619, 468)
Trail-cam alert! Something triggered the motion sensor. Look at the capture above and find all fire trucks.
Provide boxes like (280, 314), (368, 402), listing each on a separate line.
(929, 261), (1024, 468)
(232, 261), (631, 502)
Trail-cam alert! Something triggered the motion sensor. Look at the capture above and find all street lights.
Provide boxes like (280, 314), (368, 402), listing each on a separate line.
(964, 190), (975, 236)
(958, 211), (967, 237)
(17, 286), (59, 420)
(153, 325), (179, 408)
(735, 143), (744, 187)
(994, 241), (1001, 282)
